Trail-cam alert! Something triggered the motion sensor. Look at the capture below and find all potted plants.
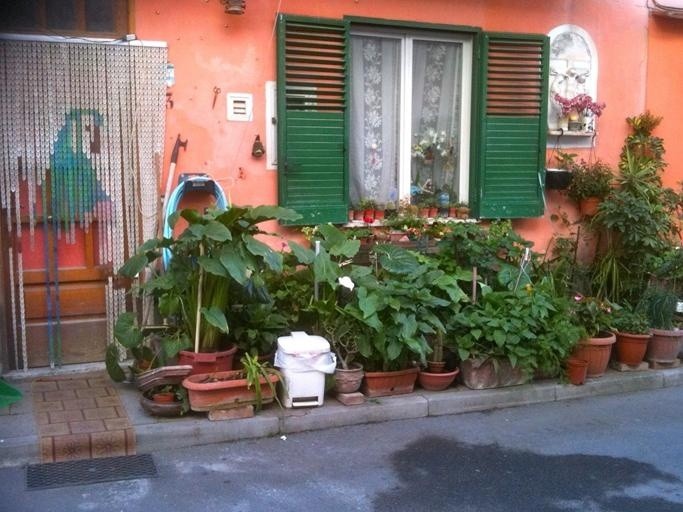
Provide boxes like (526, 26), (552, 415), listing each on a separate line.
(102, 111), (683, 416)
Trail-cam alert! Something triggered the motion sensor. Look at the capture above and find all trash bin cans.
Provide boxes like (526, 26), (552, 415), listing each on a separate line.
(276, 331), (330, 409)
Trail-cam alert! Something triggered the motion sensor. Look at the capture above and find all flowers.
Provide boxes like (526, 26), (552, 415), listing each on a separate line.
(555, 92), (602, 120)
